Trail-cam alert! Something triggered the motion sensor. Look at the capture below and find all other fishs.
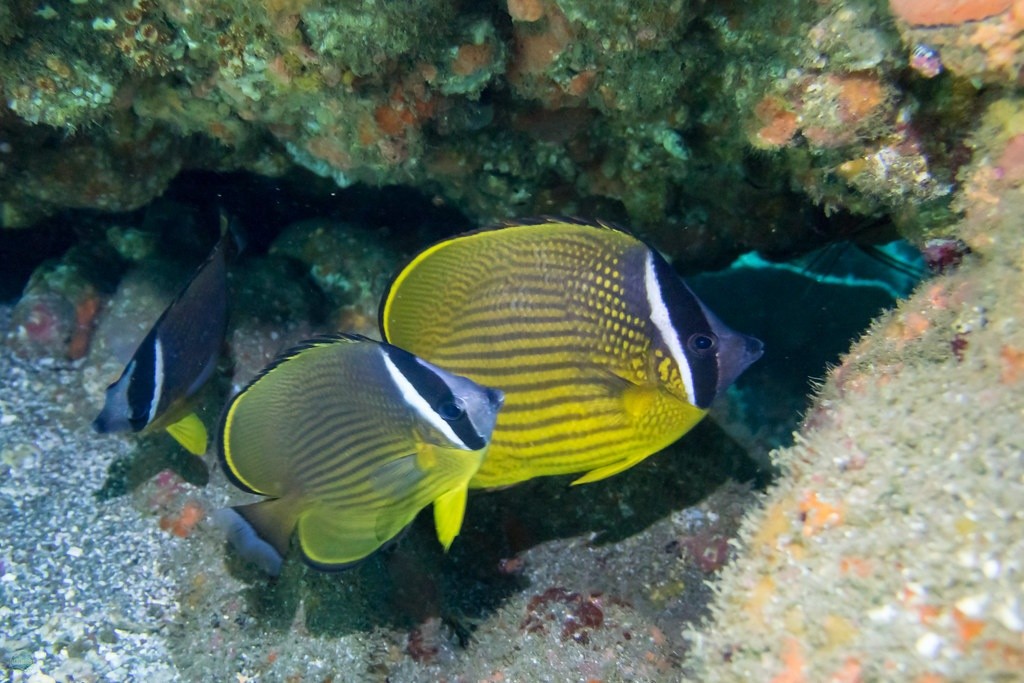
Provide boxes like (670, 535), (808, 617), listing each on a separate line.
(374, 216), (766, 493)
(203, 331), (506, 580)
(90, 211), (244, 436)
